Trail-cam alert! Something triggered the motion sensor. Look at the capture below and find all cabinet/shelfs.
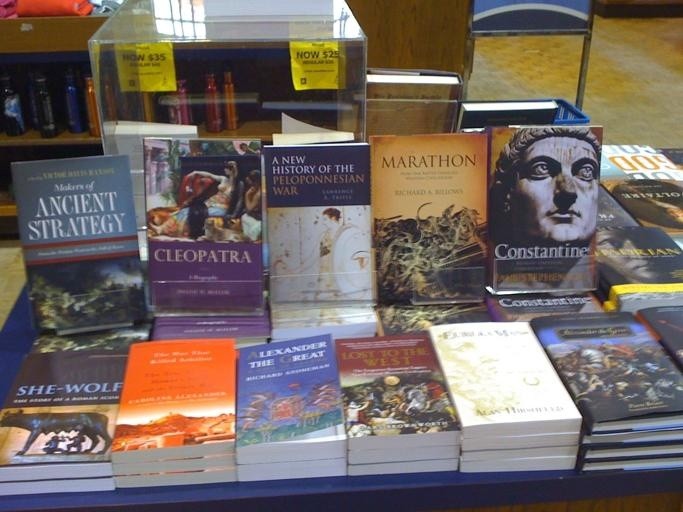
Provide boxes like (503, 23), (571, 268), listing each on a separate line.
(0, 0), (367, 218)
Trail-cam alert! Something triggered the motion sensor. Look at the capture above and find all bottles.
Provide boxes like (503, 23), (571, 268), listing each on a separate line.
(202, 71), (224, 133)
(218, 68), (241, 131)
(0, 71), (192, 137)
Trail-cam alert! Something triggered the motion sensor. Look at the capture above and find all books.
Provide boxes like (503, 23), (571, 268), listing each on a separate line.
(2, 115), (683, 497)
